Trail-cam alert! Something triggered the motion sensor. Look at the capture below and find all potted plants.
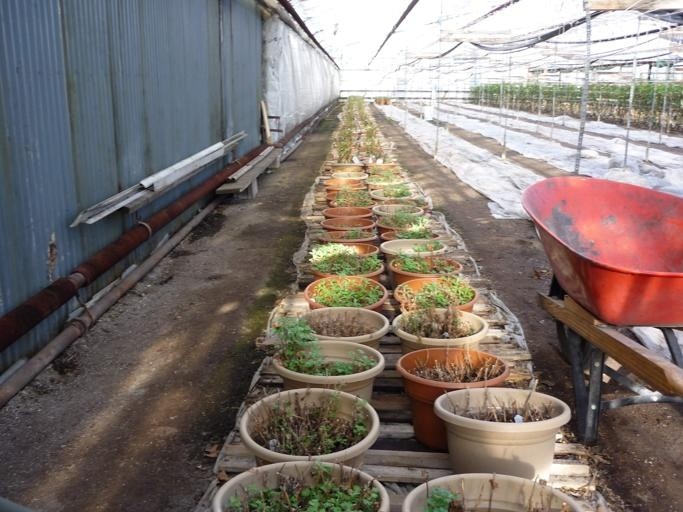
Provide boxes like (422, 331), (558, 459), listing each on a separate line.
(212, 95), (588, 512)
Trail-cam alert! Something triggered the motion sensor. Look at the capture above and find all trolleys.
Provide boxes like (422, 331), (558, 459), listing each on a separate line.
(522, 175), (683, 444)
